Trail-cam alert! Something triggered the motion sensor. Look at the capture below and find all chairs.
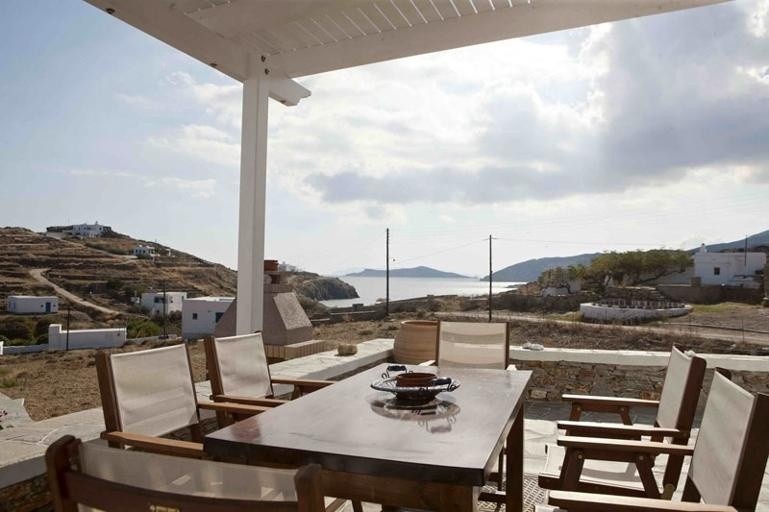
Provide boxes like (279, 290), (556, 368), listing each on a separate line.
(204, 327), (340, 412)
(43, 433), (331, 512)
(538, 341), (707, 496)
(539, 365), (767, 511)
(414, 319), (512, 489)
(93, 340), (273, 458)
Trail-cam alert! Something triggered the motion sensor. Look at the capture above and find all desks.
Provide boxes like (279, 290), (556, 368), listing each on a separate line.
(201, 360), (536, 512)
